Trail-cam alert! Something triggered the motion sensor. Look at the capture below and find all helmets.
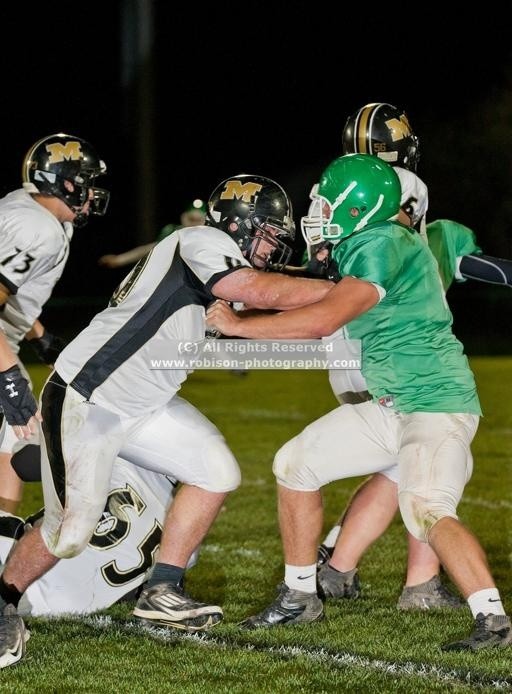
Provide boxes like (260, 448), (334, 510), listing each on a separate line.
(20, 133), (114, 229)
(342, 103), (423, 170)
(203, 172), (298, 275)
(300, 153), (402, 262)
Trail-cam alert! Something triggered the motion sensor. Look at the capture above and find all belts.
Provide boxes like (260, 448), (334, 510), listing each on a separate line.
(339, 389), (373, 405)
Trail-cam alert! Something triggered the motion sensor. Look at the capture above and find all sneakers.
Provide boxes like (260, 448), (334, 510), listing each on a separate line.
(235, 580), (327, 632)
(391, 574), (468, 611)
(316, 556), (364, 598)
(130, 583), (225, 634)
(439, 611), (512, 653)
(0, 593), (31, 671)
